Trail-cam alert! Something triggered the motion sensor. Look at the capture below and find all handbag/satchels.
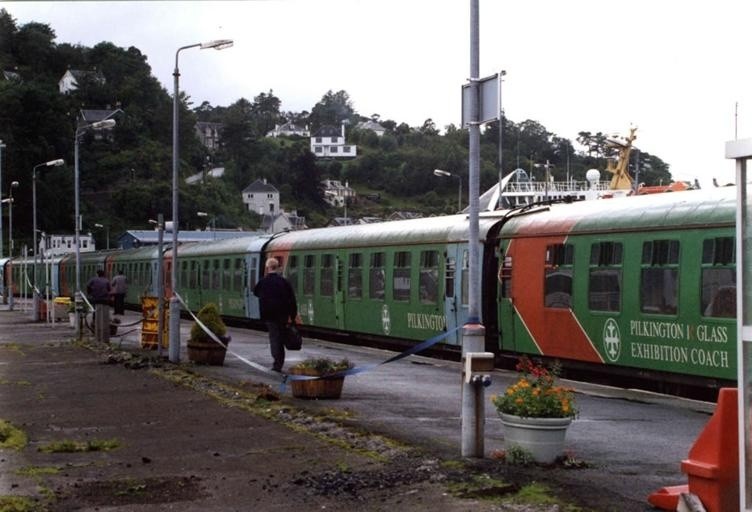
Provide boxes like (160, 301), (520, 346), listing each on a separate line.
(287, 320), (302, 350)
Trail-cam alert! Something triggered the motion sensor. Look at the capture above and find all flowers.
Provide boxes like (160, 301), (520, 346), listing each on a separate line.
(488, 353), (582, 420)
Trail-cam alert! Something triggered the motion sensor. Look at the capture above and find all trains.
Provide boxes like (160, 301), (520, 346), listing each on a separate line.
(0, 182), (751, 394)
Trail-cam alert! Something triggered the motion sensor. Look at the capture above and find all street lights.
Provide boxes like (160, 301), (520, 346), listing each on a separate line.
(32, 158), (63, 323)
(1, 196), (14, 206)
(165, 39), (237, 365)
(8, 180), (19, 311)
(74, 119), (117, 340)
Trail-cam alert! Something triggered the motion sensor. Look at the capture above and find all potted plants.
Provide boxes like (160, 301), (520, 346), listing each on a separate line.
(287, 357), (355, 398)
(187, 303), (232, 365)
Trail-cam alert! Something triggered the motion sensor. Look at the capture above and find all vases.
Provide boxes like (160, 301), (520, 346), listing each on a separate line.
(496, 409), (572, 464)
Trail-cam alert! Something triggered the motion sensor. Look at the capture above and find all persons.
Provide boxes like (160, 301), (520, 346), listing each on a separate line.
(112, 270), (128, 315)
(86, 270), (111, 328)
(253, 257), (297, 371)
